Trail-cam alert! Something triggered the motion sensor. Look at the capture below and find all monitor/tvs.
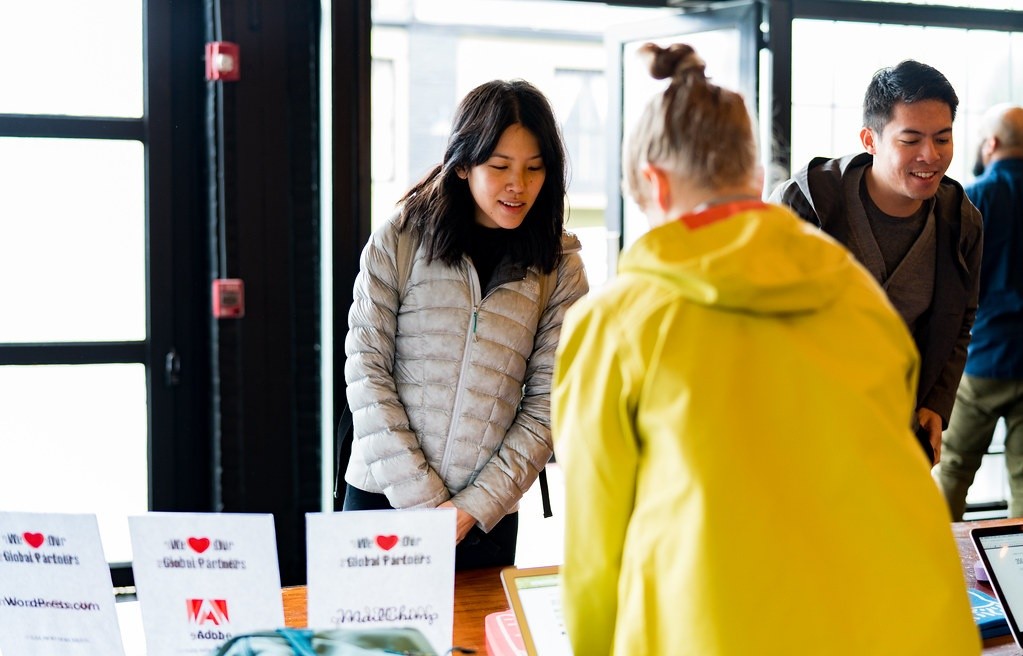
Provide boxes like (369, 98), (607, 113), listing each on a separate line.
(971, 523), (1023, 652)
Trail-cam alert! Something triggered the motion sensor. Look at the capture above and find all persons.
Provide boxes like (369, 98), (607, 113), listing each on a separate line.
(764, 58), (984, 471)
(548, 35), (988, 656)
(341, 78), (590, 579)
(931, 102), (1023, 523)
